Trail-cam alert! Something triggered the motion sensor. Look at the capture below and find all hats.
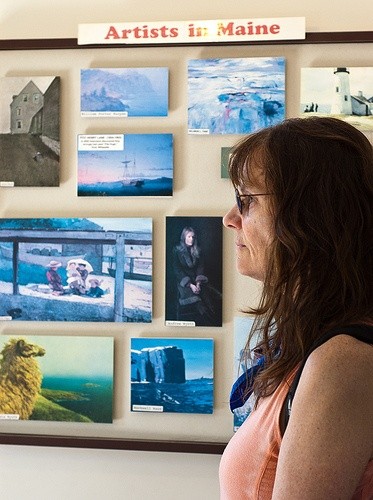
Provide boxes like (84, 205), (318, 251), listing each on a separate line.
(89, 278), (101, 286)
(47, 261), (62, 267)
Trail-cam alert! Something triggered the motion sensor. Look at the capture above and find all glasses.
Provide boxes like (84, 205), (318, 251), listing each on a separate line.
(235, 188), (275, 215)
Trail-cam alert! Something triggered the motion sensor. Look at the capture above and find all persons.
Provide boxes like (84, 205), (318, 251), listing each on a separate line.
(46, 258), (104, 298)
(219, 116), (373, 500)
(170, 224), (221, 326)
